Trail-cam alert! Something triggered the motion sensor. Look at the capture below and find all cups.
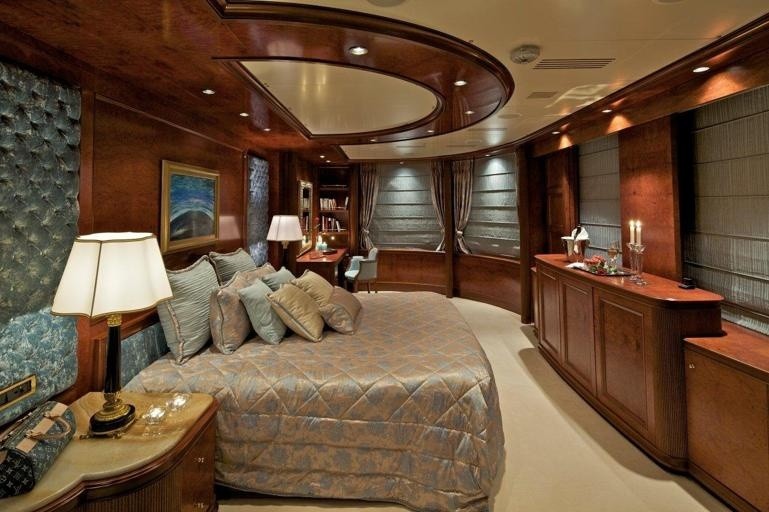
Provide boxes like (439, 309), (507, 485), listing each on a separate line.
(140, 392), (191, 440)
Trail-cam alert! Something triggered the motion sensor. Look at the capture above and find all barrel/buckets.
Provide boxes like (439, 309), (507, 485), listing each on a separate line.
(561, 236), (591, 263)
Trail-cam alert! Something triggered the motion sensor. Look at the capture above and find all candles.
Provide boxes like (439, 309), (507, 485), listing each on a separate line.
(629, 226), (634, 243)
(636, 228), (641, 244)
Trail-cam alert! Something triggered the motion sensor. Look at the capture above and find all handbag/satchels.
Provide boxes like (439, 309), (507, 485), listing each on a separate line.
(0, 401), (76, 499)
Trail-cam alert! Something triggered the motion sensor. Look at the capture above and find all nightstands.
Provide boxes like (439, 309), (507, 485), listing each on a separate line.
(0, 390), (222, 512)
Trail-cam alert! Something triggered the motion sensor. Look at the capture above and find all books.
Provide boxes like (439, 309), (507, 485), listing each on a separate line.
(320, 196), (349, 232)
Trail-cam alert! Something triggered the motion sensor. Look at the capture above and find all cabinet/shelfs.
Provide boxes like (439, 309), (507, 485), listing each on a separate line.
(317, 185), (349, 236)
(302, 208), (311, 233)
(536, 263), (561, 364)
(561, 275), (596, 397)
(684, 343), (769, 511)
(596, 285), (722, 460)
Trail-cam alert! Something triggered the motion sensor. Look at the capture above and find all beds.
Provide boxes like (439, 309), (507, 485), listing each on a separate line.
(88, 290), (506, 512)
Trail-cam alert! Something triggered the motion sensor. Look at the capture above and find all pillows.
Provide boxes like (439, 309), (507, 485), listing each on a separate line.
(155, 247), (363, 365)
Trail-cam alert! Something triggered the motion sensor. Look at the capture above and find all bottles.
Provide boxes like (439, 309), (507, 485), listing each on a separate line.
(608, 250), (617, 273)
(573, 226), (581, 240)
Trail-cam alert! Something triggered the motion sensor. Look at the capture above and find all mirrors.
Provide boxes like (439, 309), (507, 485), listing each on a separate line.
(298, 179), (313, 257)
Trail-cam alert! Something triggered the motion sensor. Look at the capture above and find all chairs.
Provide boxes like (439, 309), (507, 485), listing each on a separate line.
(343, 247), (379, 294)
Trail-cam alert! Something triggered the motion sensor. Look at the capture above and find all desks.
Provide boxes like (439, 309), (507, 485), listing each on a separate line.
(295, 248), (347, 287)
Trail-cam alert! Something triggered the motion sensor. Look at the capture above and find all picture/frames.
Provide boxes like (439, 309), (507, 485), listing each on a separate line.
(160, 158), (220, 254)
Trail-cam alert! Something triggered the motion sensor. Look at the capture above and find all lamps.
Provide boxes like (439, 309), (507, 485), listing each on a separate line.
(50, 229), (174, 439)
(265, 214), (304, 269)
